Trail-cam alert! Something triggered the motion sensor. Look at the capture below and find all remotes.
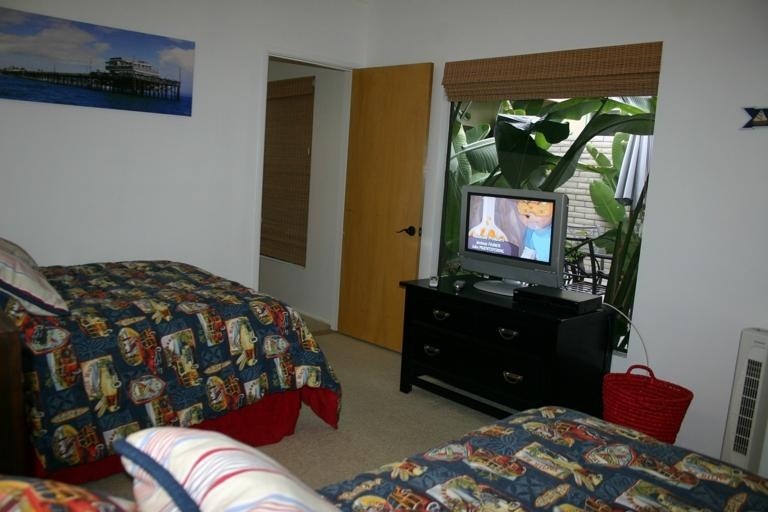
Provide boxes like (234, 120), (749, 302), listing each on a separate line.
(453, 280), (466, 293)
(430, 275), (439, 288)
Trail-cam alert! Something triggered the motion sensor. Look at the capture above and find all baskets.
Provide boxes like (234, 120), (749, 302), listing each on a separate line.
(602, 365), (693, 445)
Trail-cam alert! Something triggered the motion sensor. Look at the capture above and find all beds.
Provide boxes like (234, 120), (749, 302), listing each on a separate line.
(0, 405), (768, 512)
(0, 261), (341, 485)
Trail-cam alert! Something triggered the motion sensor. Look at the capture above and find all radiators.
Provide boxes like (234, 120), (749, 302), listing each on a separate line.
(720, 328), (768, 473)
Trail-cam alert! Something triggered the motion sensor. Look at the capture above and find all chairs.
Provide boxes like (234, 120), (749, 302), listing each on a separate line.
(562, 237), (607, 295)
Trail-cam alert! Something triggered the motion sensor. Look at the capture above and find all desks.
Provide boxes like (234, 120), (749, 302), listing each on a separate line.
(399, 274), (622, 420)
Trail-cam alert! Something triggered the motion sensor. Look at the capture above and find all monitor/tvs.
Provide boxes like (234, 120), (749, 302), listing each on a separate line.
(458, 184), (569, 297)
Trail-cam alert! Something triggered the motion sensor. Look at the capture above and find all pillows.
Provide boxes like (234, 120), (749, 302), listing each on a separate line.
(112, 426), (341, 512)
(0, 238), (71, 317)
